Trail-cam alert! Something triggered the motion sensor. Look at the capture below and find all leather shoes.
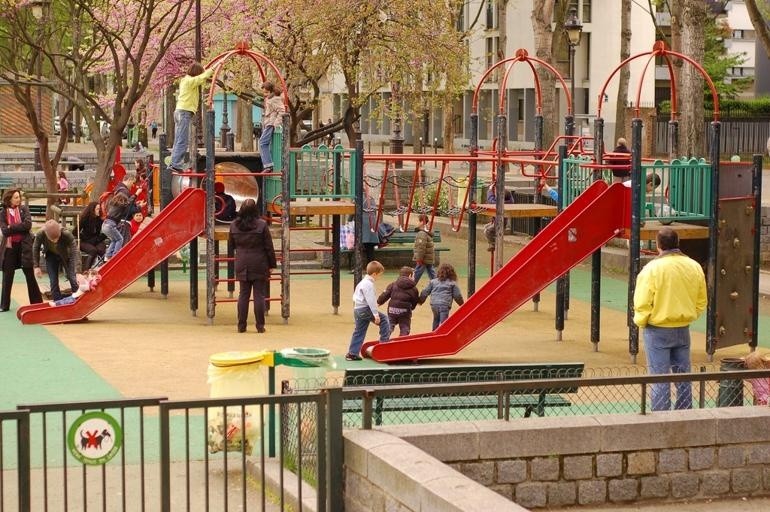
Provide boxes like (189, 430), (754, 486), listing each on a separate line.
(0, 308), (9, 312)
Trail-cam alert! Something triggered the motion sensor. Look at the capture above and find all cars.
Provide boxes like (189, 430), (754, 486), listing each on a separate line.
(53, 113), (111, 141)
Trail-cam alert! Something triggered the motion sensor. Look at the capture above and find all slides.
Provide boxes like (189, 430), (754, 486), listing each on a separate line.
(16, 188), (206, 324)
(362, 180), (632, 366)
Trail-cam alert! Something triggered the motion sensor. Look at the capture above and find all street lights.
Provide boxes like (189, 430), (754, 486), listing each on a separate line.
(562, 7), (583, 119)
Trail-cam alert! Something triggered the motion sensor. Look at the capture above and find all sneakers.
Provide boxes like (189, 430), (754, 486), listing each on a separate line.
(260, 166), (273, 173)
(238, 328), (246, 332)
(167, 164), (181, 172)
(257, 328), (265, 333)
(345, 353), (362, 361)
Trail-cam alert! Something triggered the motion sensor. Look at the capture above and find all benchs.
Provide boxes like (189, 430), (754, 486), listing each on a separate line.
(340, 227), (451, 271)
(324, 362), (584, 424)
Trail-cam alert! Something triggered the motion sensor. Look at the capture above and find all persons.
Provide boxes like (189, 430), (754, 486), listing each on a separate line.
(166, 58), (225, 173)
(226, 198), (278, 335)
(608, 137), (631, 185)
(419, 263), (464, 331)
(632, 227), (709, 411)
(376, 266), (419, 335)
(412, 215), (437, 286)
(150, 118), (159, 140)
(484, 182), (515, 252)
(257, 79), (286, 174)
(345, 261), (390, 360)
(539, 178), (581, 201)
(1, 158), (156, 333)
(621, 172), (661, 227)
(347, 187), (381, 274)
(214, 181), (236, 222)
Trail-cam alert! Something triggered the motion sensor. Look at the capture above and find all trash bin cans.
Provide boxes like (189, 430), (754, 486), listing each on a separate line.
(206, 347), (330, 457)
